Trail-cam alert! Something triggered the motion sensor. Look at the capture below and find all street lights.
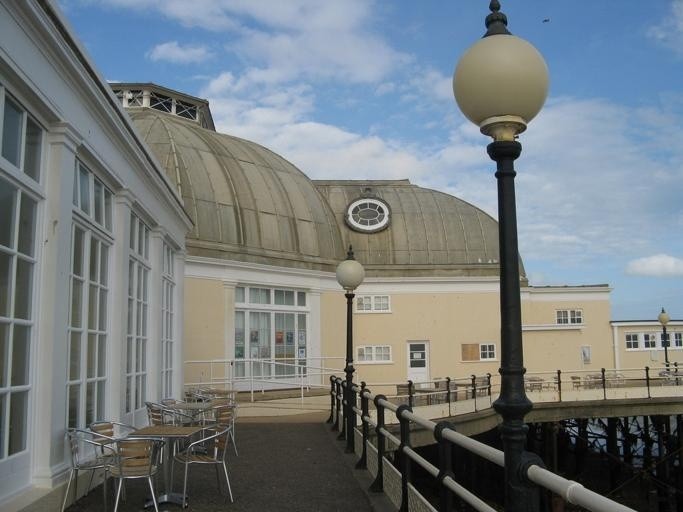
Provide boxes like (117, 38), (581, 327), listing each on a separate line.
(336, 243), (366, 454)
(456, 0), (551, 512)
(658, 307), (671, 380)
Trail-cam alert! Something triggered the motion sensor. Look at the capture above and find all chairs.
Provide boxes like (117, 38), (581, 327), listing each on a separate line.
(526, 374), (559, 393)
(569, 373), (624, 392)
(392, 376), (488, 408)
(57, 389), (239, 512)
(659, 371), (683, 387)
